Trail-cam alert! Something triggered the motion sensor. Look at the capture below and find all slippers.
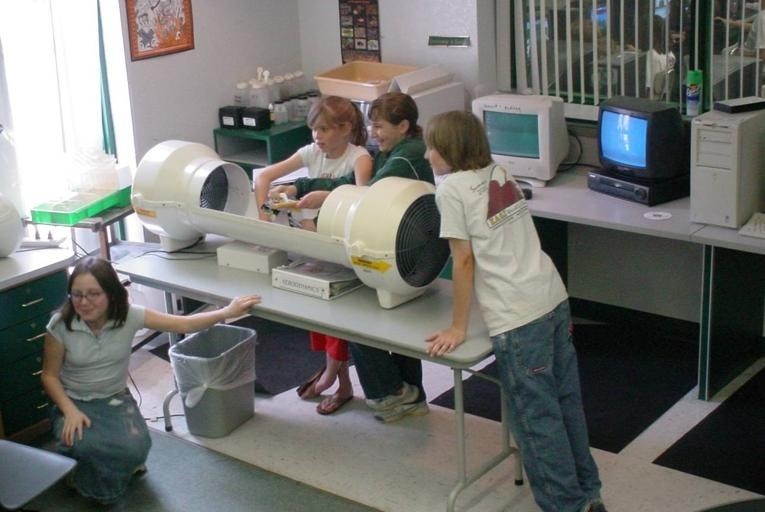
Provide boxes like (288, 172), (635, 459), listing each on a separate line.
(297, 364), (327, 397)
(317, 389), (352, 414)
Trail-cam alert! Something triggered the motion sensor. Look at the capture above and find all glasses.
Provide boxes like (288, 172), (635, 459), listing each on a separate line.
(69, 291), (104, 300)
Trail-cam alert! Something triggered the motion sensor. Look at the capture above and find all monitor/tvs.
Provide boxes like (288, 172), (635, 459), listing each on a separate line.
(470, 93), (571, 188)
(586, 94), (692, 207)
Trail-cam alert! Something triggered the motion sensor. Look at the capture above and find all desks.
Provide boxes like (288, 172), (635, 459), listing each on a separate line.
(1, 248), (77, 440)
(517, 165), (765, 401)
(113, 232), (524, 512)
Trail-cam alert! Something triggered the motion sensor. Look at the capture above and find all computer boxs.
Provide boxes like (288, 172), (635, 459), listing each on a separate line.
(690, 106), (765, 230)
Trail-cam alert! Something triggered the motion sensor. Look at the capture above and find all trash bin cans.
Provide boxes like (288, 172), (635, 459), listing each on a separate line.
(168, 322), (258, 440)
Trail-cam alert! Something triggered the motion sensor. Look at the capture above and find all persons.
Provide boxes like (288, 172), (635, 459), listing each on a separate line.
(255, 95), (373, 415)
(267, 92), (434, 424)
(423, 110), (607, 512)
(714, 0), (764, 59)
(647, 15), (675, 91)
(42, 255), (262, 504)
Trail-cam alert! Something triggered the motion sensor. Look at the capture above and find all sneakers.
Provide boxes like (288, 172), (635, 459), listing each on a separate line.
(365, 381), (419, 410)
(373, 400), (429, 424)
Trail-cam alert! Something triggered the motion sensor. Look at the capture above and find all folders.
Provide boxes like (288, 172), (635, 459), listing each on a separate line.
(270, 260), (365, 302)
